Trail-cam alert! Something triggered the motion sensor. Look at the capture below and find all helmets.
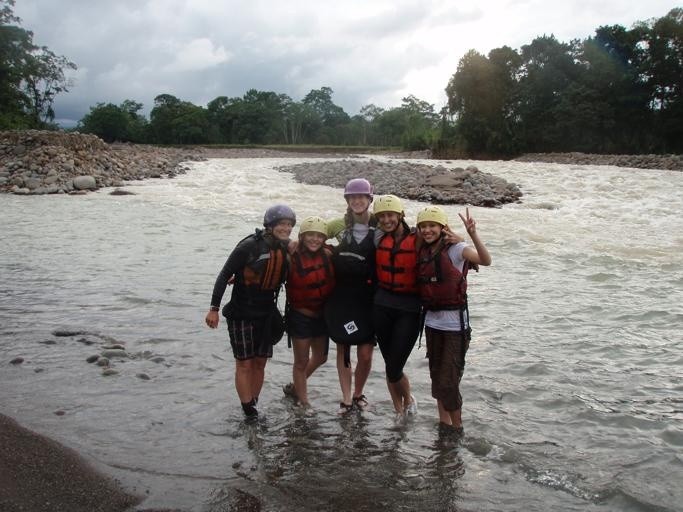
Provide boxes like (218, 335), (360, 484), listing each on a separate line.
(343, 178), (373, 203)
(373, 195), (406, 216)
(299, 217), (330, 239)
(264, 205), (296, 227)
(417, 206), (448, 226)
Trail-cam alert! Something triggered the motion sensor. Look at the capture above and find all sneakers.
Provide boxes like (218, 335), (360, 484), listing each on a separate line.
(354, 394), (371, 410)
(283, 384), (298, 402)
(440, 427), (466, 446)
(405, 395), (418, 421)
(429, 422), (452, 444)
(337, 402), (353, 418)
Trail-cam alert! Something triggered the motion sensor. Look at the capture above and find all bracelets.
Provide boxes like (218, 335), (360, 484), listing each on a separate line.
(210, 306), (220, 311)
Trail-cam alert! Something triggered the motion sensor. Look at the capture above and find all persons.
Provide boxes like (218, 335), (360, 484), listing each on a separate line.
(372, 197), (464, 413)
(206, 205), (296, 425)
(227, 216), (336, 415)
(417, 206), (492, 434)
(287, 180), (416, 415)
